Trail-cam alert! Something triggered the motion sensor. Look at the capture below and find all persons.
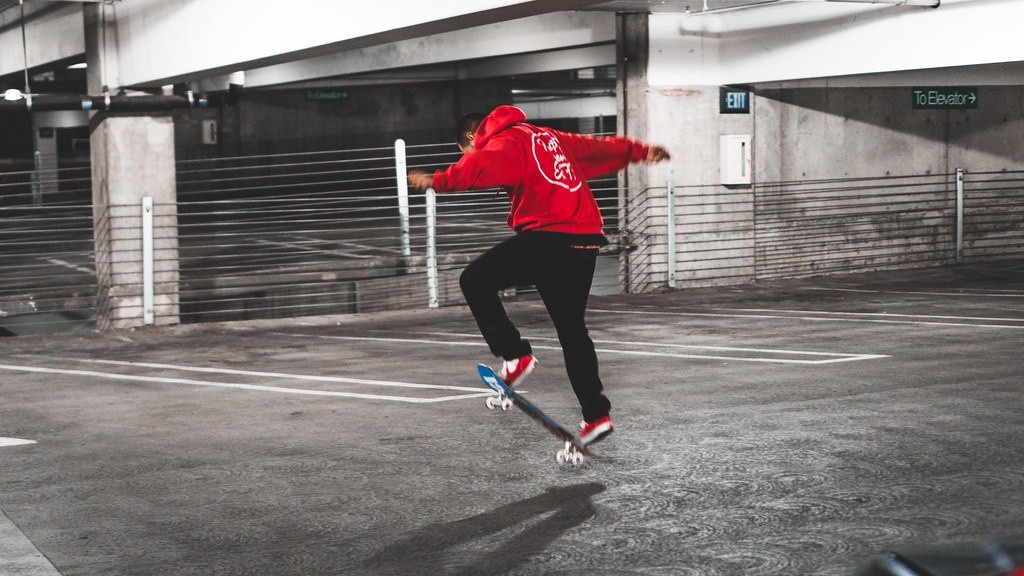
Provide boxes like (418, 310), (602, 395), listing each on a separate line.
(409, 105), (671, 446)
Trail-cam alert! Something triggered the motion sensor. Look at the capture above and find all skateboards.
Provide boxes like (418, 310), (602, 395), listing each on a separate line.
(477, 361), (601, 468)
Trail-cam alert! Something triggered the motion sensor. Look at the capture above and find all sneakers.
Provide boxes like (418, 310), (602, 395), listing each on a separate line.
(578, 416), (614, 445)
(496, 353), (538, 389)
